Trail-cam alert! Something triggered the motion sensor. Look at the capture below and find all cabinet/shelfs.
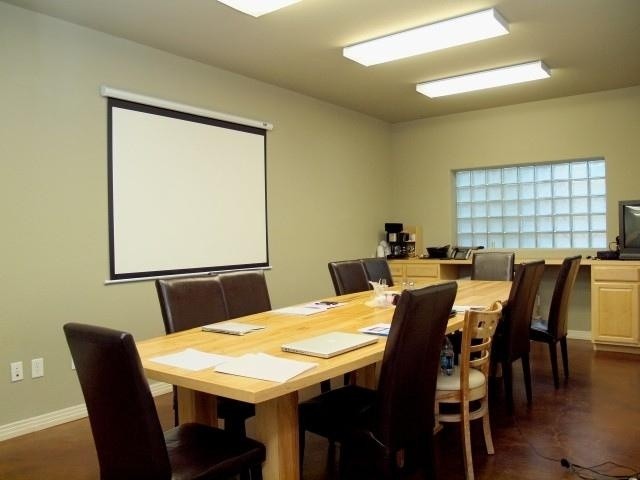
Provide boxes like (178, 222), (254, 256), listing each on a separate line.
(591, 261), (640, 354)
(386, 260), (458, 285)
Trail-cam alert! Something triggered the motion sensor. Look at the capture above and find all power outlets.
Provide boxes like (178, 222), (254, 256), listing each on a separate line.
(31, 358), (44, 379)
(10, 361), (24, 382)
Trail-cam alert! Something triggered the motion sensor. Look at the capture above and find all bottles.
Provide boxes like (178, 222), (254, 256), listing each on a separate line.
(440, 336), (454, 377)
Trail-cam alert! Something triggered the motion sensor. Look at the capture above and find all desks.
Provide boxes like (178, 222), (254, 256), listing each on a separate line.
(386, 248), (640, 354)
(135, 280), (513, 480)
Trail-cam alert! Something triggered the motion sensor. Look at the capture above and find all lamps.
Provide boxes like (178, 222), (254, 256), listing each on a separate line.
(414, 59), (551, 98)
(341, 9), (511, 67)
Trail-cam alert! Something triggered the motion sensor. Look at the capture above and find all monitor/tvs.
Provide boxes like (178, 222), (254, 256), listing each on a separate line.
(618, 200), (640, 260)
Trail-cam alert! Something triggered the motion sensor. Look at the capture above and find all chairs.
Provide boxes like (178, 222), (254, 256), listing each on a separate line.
(208, 269), (331, 394)
(328, 260), (371, 385)
(531, 254), (582, 390)
(155, 275), (255, 437)
(358, 258), (393, 290)
(435, 300), (502, 480)
(453, 259), (545, 416)
(471, 252), (515, 281)
(63, 322), (266, 480)
(298, 281), (458, 480)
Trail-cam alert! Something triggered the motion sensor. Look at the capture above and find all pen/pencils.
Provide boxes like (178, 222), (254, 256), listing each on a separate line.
(315, 301), (338, 306)
